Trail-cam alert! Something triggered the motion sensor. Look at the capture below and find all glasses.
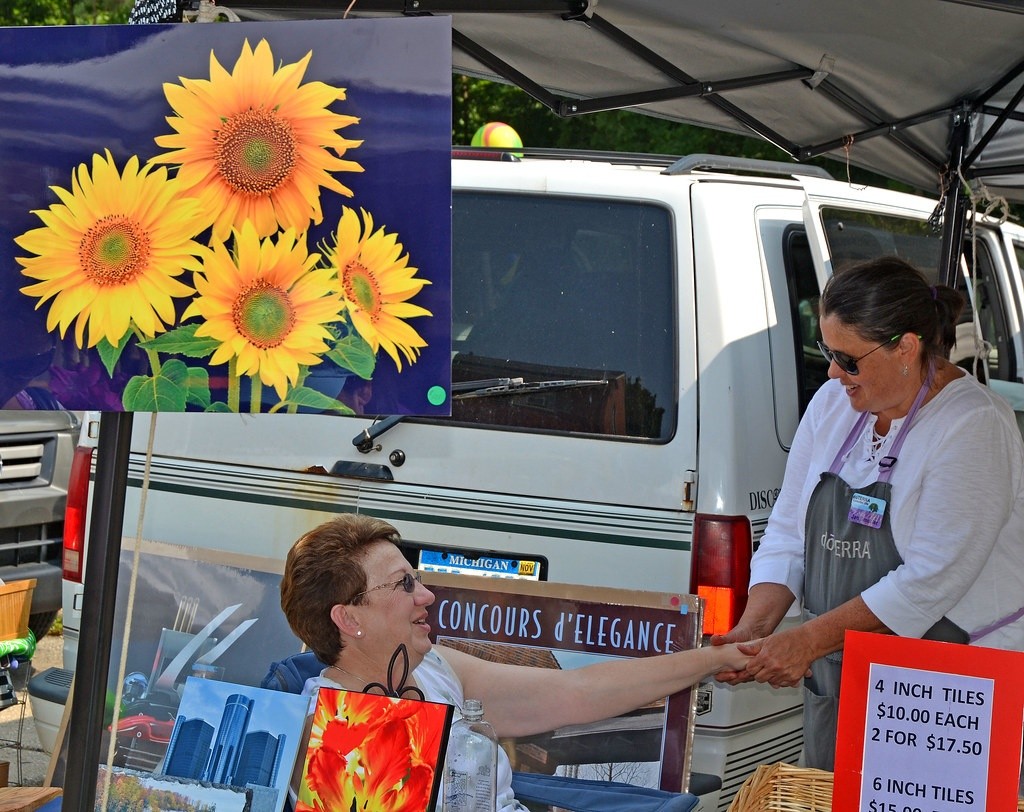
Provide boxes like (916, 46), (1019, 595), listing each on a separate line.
(346, 572), (421, 607)
(817, 334), (922, 375)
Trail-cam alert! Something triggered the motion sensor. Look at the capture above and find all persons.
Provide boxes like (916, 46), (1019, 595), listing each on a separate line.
(706, 258), (1021, 770)
(277, 512), (816, 811)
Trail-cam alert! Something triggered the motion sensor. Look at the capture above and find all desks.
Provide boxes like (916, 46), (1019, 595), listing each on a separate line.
(0, 627), (37, 787)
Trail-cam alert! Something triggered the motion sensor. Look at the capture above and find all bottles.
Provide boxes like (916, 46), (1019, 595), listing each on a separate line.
(443, 699), (499, 812)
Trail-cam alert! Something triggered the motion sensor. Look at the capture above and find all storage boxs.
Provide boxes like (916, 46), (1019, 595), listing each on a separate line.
(0, 578), (38, 642)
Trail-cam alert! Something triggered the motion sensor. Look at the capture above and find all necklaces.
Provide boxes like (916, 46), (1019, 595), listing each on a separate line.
(330, 665), (379, 695)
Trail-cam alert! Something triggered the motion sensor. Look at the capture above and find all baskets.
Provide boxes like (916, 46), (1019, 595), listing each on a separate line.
(726, 761), (834, 812)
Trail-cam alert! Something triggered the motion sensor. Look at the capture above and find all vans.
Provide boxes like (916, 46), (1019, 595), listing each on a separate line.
(25, 142), (1023, 811)
(0, 408), (81, 643)
